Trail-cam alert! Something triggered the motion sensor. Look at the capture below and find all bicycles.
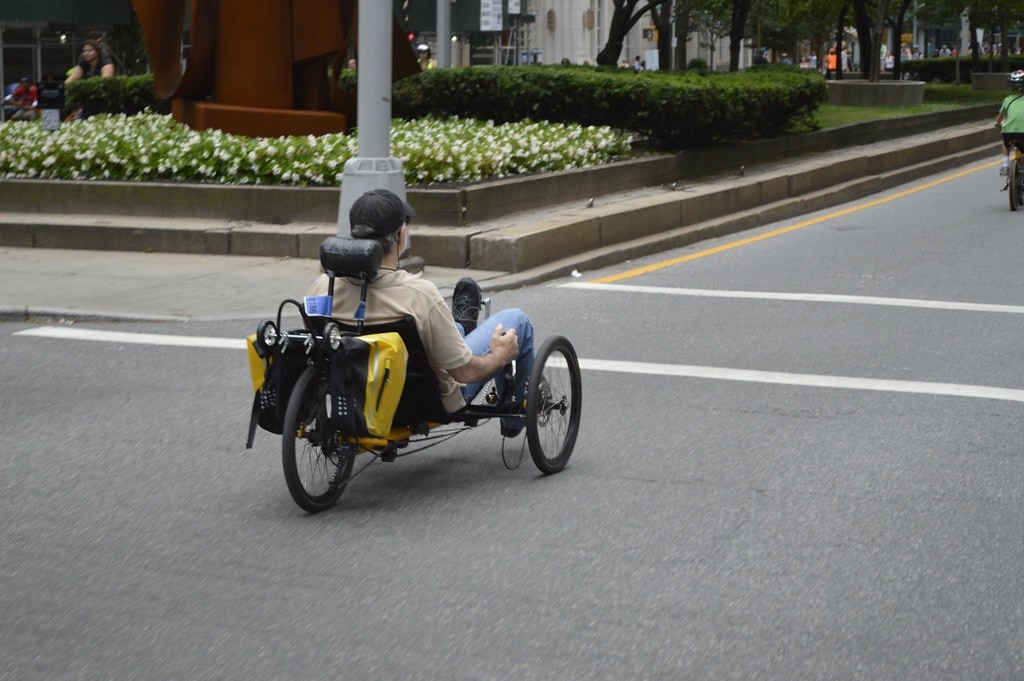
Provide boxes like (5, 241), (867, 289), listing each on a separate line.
(994, 124), (1024, 211)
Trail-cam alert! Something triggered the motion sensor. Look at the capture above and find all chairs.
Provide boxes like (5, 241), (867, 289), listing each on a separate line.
(302, 237), (460, 425)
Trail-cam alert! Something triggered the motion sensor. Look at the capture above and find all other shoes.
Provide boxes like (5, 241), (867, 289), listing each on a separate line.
(1000, 166), (1009, 175)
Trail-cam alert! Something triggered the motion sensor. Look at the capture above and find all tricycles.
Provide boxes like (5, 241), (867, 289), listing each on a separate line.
(244, 233), (583, 515)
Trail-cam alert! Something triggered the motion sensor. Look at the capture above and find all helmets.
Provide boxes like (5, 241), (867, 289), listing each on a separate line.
(1007, 70), (1024, 86)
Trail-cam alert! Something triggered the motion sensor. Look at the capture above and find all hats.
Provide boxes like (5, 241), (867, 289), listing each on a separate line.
(350, 189), (415, 239)
(829, 48), (835, 52)
(942, 45), (946, 49)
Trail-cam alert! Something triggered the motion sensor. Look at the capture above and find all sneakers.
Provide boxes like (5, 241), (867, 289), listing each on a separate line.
(501, 419), (519, 439)
(451, 278), (479, 335)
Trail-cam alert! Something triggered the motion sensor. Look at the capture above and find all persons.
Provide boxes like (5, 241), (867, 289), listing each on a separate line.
(754, 51), (768, 65)
(780, 47), (837, 80)
(879, 43), (895, 73)
(913, 44), (921, 60)
(633, 55), (646, 74)
(899, 41), (912, 61)
(617, 58), (630, 69)
(840, 47), (852, 72)
(347, 58), (356, 70)
(995, 69), (1024, 175)
(63, 30), (124, 87)
(967, 39), (1022, 56)
(415, 43), (437, 71)
(939, 44), (952, 59)
(927, 37), (938, 59)
(0, 76), (40, 122)
(305, 190), (548, 439)
